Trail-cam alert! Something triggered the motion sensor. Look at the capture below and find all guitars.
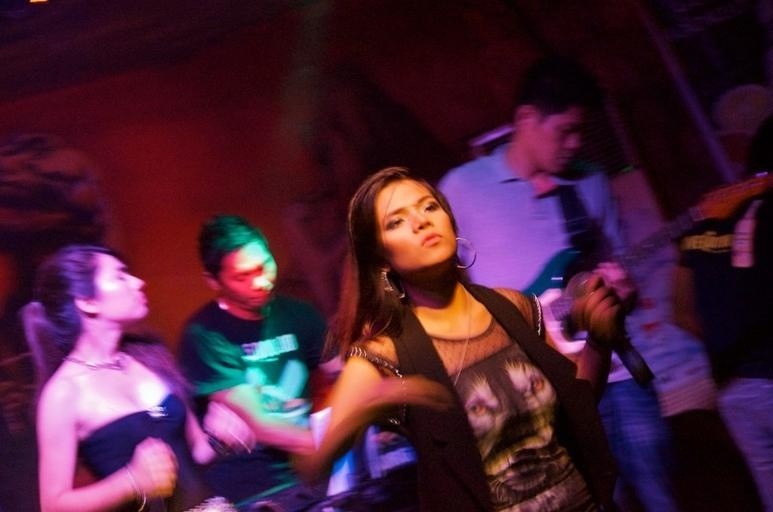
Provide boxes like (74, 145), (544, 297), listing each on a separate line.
(538, 171), (773, 363)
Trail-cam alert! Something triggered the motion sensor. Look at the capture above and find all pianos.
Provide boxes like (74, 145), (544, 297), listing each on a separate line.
(231, 441), (418, 508)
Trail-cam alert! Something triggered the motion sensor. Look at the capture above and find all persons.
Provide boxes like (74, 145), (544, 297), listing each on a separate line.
(33, 245), (256, 512)
(181, 217), (338, 505)
(292, 168), (625, 512)
(439, 57), (667, 512)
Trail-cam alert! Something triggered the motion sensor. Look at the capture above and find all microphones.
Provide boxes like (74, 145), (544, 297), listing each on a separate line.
(567, 270), (657, 389)
